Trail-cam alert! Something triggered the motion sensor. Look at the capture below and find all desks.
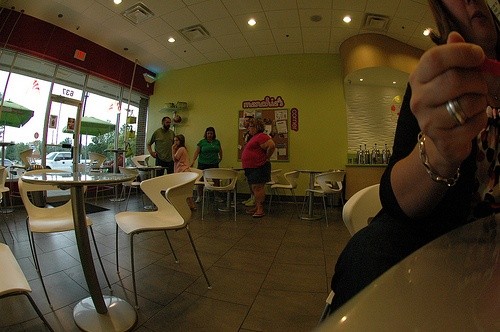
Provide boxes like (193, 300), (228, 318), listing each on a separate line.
(107, 173), (127, 203)
(297, 170), (325, 220)
(21, 171), (138, 332)
(137, 166), (170, 210)
(217, 169), (245, 212)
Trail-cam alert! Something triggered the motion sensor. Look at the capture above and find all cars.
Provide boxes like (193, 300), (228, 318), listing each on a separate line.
(0, 158), (32, 178)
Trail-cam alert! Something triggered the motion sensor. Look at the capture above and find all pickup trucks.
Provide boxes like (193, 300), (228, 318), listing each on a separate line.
(31, 151), (103, 174)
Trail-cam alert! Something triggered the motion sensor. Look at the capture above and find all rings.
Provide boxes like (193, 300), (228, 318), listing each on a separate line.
(446, 99), (469, 126)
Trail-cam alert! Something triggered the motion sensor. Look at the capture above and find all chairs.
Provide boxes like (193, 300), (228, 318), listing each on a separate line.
(130, 154), (383, 238)
(0, 165), (15, 244)
(0, 243), (56, 332)
(114, 172), (214, 311)
(18, 169), (113, 306)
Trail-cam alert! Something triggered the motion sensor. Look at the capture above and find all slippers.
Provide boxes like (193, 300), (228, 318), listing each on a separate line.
(252, 212), (265, 217)
(249, 208), (257, 213)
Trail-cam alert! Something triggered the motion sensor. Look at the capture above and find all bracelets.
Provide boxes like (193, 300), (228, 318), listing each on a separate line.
(417, 131), (460, 186)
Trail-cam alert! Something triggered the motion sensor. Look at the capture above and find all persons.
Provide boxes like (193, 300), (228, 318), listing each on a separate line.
(241, 118), (276, 218)
(147, 117), (176, 177)
(102, 152), (124, 173)
(172, 134), (197, 210)
(326, 0), (500, 314)
(190, 127), (224, 203)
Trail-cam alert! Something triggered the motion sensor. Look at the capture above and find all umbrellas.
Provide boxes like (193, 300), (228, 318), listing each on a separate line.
(63, 117), (116, 167)
(0, 100), (34, 141)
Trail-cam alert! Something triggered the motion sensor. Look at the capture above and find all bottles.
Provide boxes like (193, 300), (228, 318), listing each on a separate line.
(357, 144), (391, 164)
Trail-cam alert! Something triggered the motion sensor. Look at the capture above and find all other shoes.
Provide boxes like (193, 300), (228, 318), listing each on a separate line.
(215, 197), (223, 203)
(188, 204), (194, 209)
(242, 199), (251, 204)
(195, 197), (202, 202)
(245, 200), (256, 207)
